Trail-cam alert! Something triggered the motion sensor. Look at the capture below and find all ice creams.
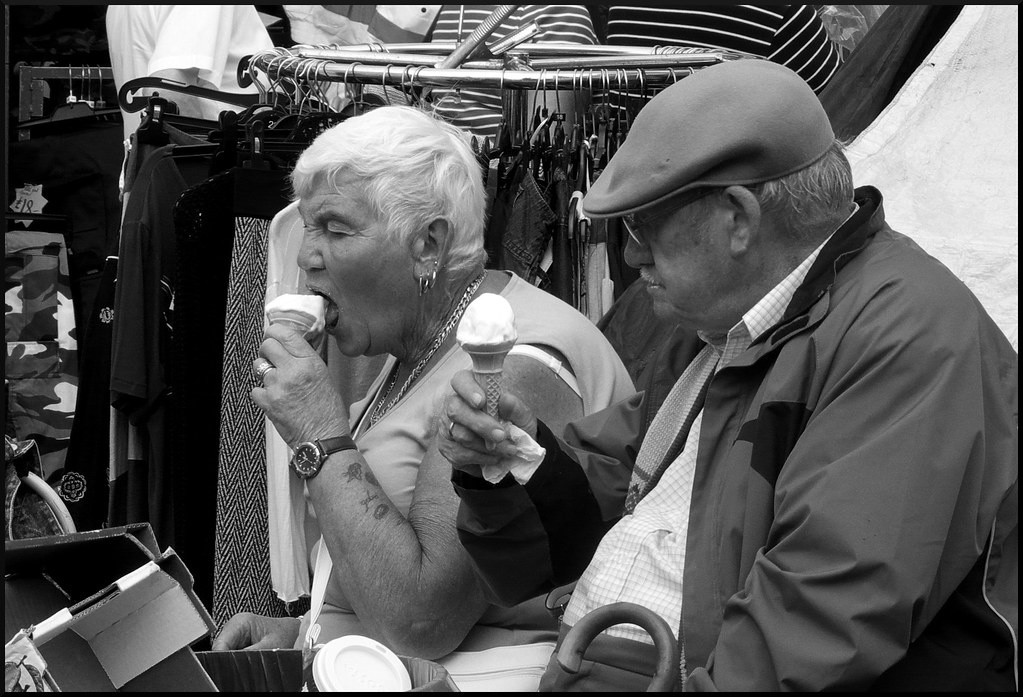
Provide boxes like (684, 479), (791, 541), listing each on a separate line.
(455, 292), (519, 451)
(266, 293), (326, 343)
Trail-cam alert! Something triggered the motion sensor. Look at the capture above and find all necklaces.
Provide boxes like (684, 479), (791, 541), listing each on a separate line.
(370, 270), (488, 427)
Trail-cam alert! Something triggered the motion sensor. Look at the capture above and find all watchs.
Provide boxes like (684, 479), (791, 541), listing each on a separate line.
(289, 434), (357, 479)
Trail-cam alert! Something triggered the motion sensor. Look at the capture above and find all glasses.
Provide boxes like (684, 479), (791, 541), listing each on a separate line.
(623, 186), (760, 241)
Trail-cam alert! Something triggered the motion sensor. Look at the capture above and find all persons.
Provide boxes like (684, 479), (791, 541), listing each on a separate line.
(606, 5), (845, 96)
(213, 105), (638, 659)
(105, 5), (601, 194)
(435, 59), (1018, 691)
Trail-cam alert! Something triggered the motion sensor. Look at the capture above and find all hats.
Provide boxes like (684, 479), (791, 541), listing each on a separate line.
(582, 60), (838, 218)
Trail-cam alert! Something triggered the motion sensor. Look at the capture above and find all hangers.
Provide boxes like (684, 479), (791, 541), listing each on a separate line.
(65, 39), (731, 259)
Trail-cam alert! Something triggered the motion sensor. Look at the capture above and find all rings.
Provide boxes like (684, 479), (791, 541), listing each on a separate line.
(255, 361), (273, 379)
(448, 421), (456, 437)
